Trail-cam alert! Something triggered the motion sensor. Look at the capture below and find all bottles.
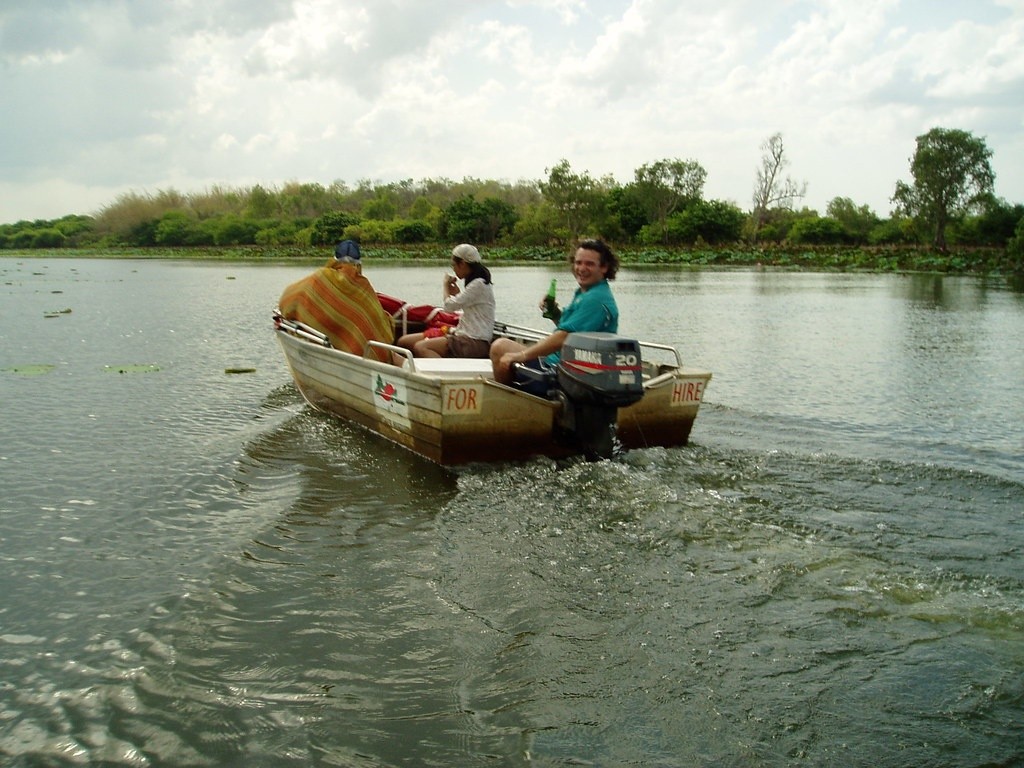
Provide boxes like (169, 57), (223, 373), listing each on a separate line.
(543, 278), (558, 319)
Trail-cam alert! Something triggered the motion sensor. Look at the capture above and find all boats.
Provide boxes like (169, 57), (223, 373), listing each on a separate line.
(269, 294), (713, 470)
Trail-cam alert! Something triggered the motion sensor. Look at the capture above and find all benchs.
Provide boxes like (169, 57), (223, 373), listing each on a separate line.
(401, 356), (493, 379)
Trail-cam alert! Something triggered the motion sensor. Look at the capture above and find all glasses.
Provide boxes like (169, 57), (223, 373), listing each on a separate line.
(584, 238), (596, 243)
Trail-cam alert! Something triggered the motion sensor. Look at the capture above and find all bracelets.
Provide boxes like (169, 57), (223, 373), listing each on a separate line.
(522, 351), (526, 362)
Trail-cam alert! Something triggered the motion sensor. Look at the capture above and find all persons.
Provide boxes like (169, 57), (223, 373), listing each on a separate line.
(490, 240), (619, 388)
(397, 243), (495, 358)
(279, 240), (404, 367)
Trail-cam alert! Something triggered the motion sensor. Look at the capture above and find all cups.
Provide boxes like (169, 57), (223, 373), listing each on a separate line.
(449, 276), (457, 285)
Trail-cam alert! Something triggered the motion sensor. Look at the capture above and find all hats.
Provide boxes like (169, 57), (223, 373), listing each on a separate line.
(334, 240), (361, 265)
(452, 244), (482, 263)
